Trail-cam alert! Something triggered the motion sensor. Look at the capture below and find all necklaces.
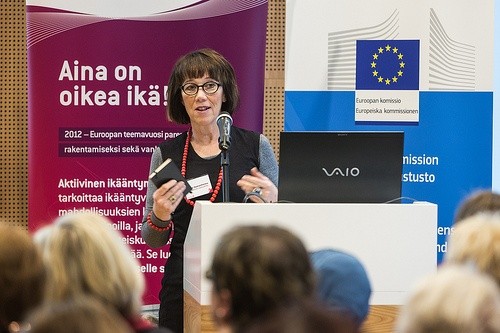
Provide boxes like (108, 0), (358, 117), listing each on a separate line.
(179, 126), (224, 208)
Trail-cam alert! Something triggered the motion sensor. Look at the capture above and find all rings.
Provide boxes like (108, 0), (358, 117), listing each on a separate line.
(169, 196), (176, 204)
(252, 187), (262, 196)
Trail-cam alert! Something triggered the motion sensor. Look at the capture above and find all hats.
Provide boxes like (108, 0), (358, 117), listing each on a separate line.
(306, 249), (373, 319)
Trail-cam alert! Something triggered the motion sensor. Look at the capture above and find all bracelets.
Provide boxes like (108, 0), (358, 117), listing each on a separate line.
(146, 211), (173, 231)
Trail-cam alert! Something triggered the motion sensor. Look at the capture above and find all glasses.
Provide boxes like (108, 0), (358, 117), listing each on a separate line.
(180, 81), (224, 95)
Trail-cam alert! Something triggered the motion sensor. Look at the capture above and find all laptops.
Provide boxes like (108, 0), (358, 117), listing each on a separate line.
(277, 130), (405, 204)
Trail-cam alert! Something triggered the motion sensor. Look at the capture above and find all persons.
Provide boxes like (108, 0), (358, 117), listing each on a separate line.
(443, 210), (500, 279)
(388, 266), (499, 332)
(30, 210), (174, 333)
(454, 189), (500, 223)
(142, 46), (280, 331)
(207, 223), (316, 333)
(303, 245), (371, 333)
(0, 221), (50, 333)
(31, 294), (132, 333)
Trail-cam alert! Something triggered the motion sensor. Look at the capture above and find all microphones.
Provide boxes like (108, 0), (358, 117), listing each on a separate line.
(215, 111), (233, 151)
